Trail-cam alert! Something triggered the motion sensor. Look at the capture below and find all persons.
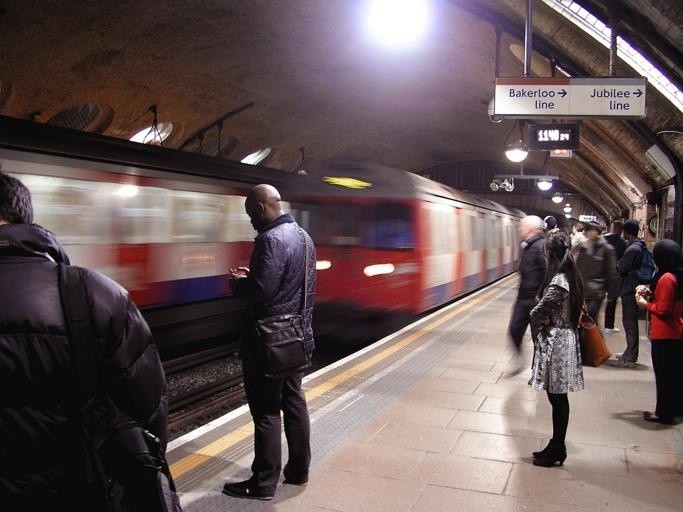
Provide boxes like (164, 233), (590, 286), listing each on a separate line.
(634, 239), (682, 426)
(502, 213), (654, 379)
(526, 230), (585, 467)
(220, 182), (318, 500)
(0, 169), (172, 512)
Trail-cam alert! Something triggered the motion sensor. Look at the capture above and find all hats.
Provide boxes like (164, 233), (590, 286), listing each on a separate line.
(583, 221), (602, 235)
(623, 220), (639, 235)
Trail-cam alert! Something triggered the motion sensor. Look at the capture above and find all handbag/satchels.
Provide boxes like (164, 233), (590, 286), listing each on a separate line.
(247, 314), (307, 378)
(578, 313), (611, 368)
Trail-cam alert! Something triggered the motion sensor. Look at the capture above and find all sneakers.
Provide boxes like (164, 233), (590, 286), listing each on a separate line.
(612, 352), (636, 368)
(604, 326), (620, 332)
(643, 411), (667, 421)
(222, 481), (272, 500)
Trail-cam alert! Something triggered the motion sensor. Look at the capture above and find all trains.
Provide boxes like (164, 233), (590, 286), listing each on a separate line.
(273, 154), (532, 343)
(0, 114), (311, 339)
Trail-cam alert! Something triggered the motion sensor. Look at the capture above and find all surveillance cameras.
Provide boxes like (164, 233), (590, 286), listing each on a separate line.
(487, 97), (504, 123)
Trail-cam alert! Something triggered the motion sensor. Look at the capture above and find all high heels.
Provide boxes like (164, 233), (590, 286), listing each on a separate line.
(533, 440), (566, 466)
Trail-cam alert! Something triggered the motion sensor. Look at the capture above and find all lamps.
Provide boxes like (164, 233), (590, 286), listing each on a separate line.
(503, 119), (563, 204)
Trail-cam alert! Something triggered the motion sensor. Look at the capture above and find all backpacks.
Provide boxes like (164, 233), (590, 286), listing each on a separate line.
(58, 262), (183, 512)
(631, 243), (654, 283)
